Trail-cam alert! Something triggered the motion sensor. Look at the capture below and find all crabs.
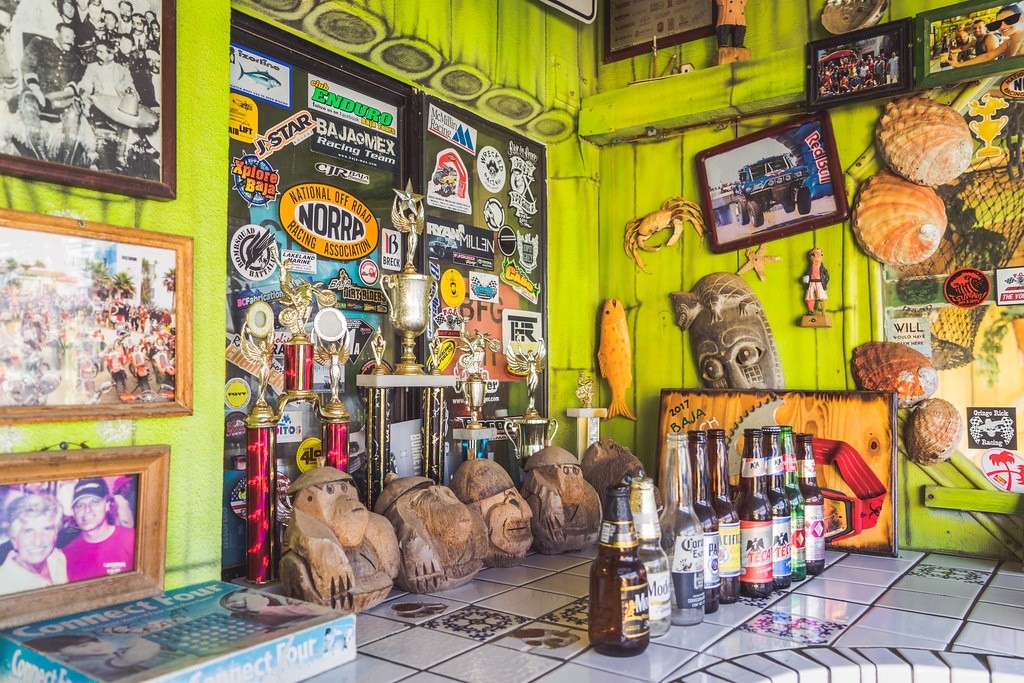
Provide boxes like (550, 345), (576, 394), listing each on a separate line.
(623, 195), (708, 274)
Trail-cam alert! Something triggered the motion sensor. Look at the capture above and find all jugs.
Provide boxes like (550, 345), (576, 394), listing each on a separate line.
(116, 88), (138, 116)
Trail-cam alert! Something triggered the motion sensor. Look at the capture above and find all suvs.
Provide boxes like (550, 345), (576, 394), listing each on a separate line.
(731, 152), (814, 230)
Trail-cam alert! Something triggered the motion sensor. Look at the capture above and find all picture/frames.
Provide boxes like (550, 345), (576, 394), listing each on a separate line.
(605, 0), (716, 64)
(1, 446), (172, 622)
(807, 18), (914, 110)
(232, 8), (546, 455)
(697, 111), (852, 254)
(0, 0), (180, 199)
(914, 1), (1023, 92)
(0, 207), (193, 426)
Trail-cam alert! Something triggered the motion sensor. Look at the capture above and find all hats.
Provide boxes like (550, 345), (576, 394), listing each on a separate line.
(71, 477), (109, 505)
(114, 477), (134, 490)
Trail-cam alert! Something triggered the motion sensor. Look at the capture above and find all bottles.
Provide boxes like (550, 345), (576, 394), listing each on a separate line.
(687, 430), (719, 614)
(708, 429), (741, 604)
(796, 434), (825, 574)
(762, 426), (792, 588)
(628, 476), (671, 638)
(736, 428), (773, 598)
(589, 485), (650, 658)
(659, 433), (705, 625)
(779, 427), (807, 582)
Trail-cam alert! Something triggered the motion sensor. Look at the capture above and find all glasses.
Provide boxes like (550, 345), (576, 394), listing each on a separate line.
(985, 13), (1021, 32)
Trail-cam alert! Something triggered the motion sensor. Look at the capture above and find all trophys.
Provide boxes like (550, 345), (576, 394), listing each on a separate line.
(239, 247), (350, 582)
(356, 179), (457, 512)
(565, 372), (608, 465)
(503, 337), (558, 469)
(453, 329), (496, 461)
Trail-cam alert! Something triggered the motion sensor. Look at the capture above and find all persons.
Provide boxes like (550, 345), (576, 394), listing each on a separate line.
(818, 51), (900, 99)
(0, 0), (163, 184)
(23, 636), (178, 683)
(941, 3), (1024, 68)
(1, 290), (179, 336)
(715, 0), (747, 48)
(0, 473), (137, 595)
(803, 247), (830, 317)
(219, 587), (331, 623)
(326, 626), (353, 653)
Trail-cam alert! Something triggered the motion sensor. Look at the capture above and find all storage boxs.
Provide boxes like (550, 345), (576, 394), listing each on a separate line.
(0, 580), (356, 682)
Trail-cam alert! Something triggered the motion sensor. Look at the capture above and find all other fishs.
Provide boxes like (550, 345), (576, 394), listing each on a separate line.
(598, 299), (638, 422)
